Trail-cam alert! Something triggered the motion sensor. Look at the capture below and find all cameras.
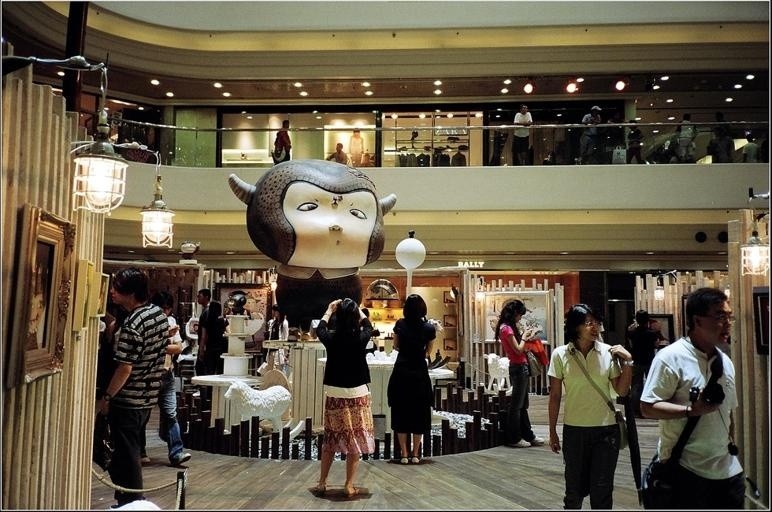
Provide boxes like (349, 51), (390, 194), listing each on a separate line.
(701, 384), (725, 405)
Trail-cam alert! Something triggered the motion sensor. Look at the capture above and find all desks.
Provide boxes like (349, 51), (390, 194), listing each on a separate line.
(191, 374), (266, 435)
(315, 355), (465, 434)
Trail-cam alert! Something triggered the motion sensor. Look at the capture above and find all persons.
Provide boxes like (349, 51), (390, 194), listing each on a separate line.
(386, 293), (437, 465)
(760, 130), (769, 163)
(315, 297), (377, 499)
(325, 142), (348, 166)
(195, 288), (289, 401)
(272, 118), (292, 166)
(623, 119), (650, 164)
(711, 111), (734, 163)
(493, 299), (545, 449)
(673, 112), (697, 163)
(741, 134), (761, 163)
(511, 103), (535, 166)
(574, 105), (604, 164)
(96, 268), (191, 509)
(648, 317), (665, 346)
(626, 309), (661, 418)
(349, 127), (366, 168)
(639, 285), (746, 511)
(544, 302), (635, 510)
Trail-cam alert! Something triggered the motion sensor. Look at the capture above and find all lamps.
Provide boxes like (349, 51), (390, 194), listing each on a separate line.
(70, 139), (176, 249)
(739, 190), (770, 275)
(655, 270), (679, 300)
(2, 55), (129, 215)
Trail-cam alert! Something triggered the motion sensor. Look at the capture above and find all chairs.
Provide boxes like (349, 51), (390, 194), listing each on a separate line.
(258, 370), (294, 450)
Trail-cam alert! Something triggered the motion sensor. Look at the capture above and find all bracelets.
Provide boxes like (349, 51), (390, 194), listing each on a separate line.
(520, 337), (527, 342)
(682, 404), (691, 418)
(323, 312), (333, 318)
(623, 358), (635, 368)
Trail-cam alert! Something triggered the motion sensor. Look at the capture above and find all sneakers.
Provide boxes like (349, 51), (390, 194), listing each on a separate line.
(172, 453), (191, 466)
(401, 456), (419, 464)
(514, 436), (545, 447)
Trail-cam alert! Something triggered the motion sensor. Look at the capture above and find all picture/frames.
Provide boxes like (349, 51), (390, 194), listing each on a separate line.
(71, 259), (110, 332)
(480, 289), (553, 345)
(444, 291), (457, 351)
(648, 314), (675, 349)
(6, 202), (76, 390)
(753, 287), (771, 358)
(682, 295), (730, 345)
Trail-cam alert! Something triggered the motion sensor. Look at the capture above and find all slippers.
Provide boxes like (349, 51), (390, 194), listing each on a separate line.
(345, 487), (359, 497)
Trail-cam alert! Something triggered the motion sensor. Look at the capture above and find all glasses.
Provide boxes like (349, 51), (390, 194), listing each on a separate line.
(585, 321), (601, 327)
(700, 313), (737, 323)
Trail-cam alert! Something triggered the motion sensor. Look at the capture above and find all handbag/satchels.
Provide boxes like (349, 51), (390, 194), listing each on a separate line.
(640, 460), (694, 509)
(525, 339), (549, 377)
(615, 410), (628, 450)
(257, 362), (268, 375)
